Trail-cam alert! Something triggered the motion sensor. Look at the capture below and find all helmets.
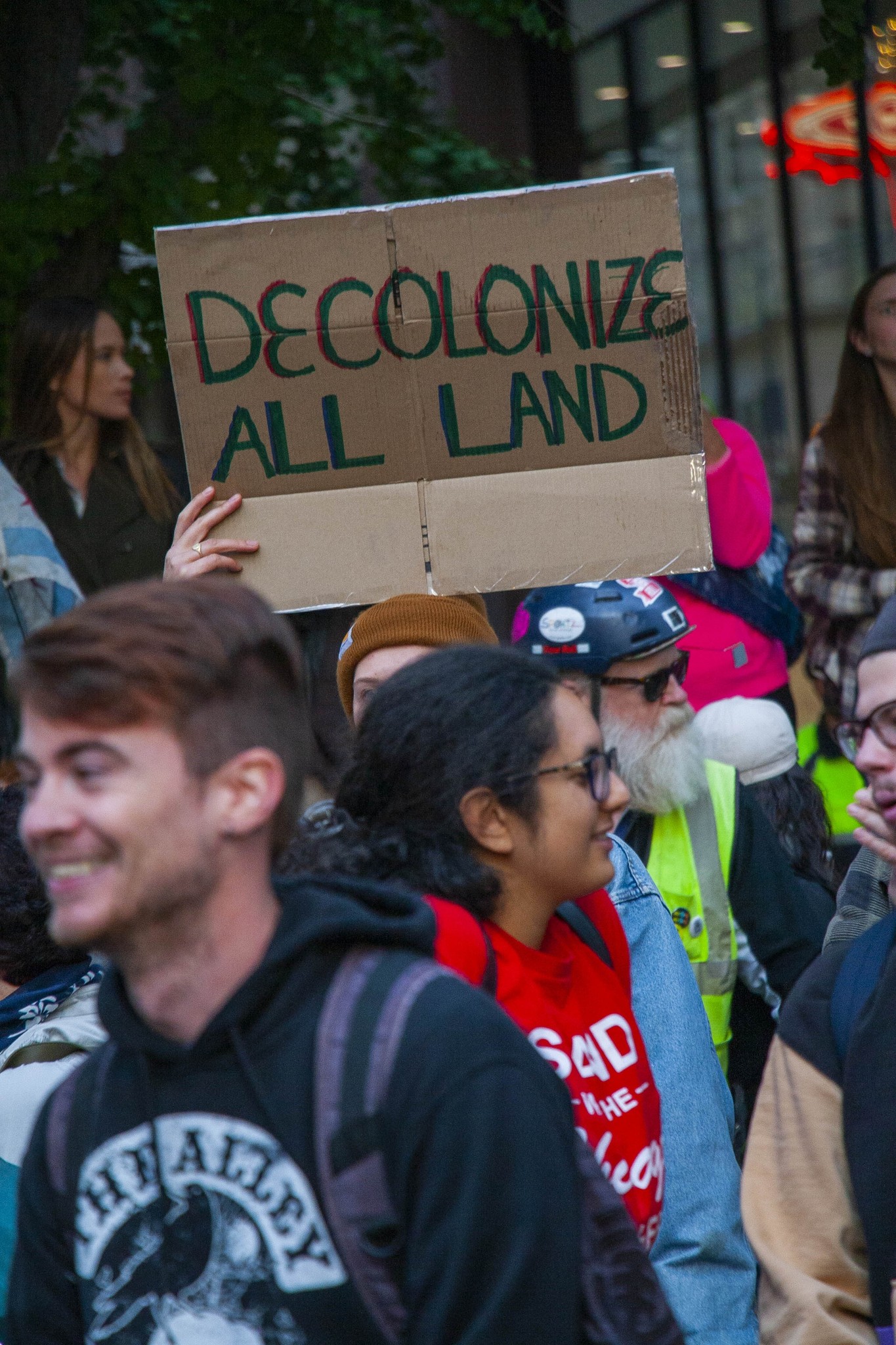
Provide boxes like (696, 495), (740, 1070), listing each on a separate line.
(507, 577), (699, 680)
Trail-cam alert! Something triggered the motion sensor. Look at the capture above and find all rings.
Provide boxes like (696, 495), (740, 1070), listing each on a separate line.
(192, 543), (203, 561)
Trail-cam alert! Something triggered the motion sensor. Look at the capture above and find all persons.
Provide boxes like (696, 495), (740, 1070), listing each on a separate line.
(738, 598), (895, 1345)
(1, 459), (260, 710)
(288, 649), (668, 1254)
(0, 578), (692, 1344)
(510, 578), (829, 1171)
(511, 419), (798, 746)
(1, 781), (110, 1342)
(4, 292), (189, 604)
(692, 692), (845, 1138)
(822, 782), (896, 951)
(789, 262), (896, 760)
(334, 593), (763, 1345)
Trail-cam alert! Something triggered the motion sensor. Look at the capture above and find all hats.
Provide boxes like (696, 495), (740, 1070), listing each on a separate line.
(693, 695), (801, 789)
(336, 591), (500, 734)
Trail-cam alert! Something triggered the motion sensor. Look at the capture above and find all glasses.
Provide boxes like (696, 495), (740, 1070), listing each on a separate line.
(832, 698), (896, 765)
(594, 648), (689, 703)
(481, 746), (621, 802)
